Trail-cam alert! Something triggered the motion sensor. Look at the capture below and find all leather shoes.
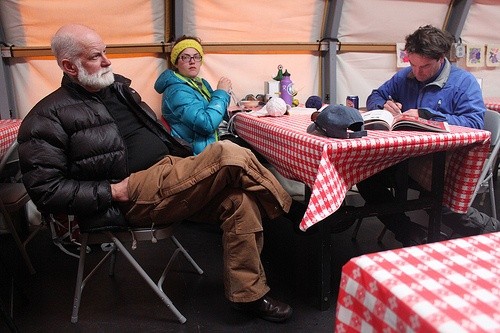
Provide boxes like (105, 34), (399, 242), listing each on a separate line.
(231, 294), (293, 321)
(293, 206), (357, 236)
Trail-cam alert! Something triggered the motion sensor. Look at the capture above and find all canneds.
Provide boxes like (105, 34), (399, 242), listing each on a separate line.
(346, 95), (358, 110)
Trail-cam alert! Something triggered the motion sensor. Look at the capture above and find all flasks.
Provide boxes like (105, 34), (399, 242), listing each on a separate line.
(279, 68), (292, 109)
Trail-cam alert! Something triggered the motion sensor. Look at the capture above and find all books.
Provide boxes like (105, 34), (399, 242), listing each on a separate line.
(361, 109), (450, 132)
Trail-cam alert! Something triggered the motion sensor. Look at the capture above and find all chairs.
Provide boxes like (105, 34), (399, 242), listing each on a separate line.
(46, 216), (205, 326)
(438, 103), (500, 235)
(0, 136), (46, 281)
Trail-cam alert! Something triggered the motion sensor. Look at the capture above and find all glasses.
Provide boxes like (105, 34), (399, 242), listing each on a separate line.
(178, 54), (202, 62)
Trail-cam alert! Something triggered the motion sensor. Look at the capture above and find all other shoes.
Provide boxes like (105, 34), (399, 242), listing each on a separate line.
(407, 229), (450, 248)
(483, 217), (500, 233)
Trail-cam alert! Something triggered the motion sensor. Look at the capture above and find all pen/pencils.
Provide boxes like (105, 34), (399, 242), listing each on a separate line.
(389, 95), (403, 114)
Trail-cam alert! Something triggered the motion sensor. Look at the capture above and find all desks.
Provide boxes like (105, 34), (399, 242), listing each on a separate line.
(333, 229), (500, 333)
(233, 106), (492, 244)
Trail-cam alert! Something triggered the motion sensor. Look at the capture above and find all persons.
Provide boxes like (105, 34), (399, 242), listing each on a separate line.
(153, 35), (270, 172)
(18, 22), (360, 323)
(355, 25), (500, 247)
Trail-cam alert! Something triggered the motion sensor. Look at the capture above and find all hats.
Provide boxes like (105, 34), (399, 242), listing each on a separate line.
(307, 104), (367, 138)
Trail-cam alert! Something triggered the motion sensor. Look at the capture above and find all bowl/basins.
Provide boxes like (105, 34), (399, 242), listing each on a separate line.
(240, 101), (260, 108)
